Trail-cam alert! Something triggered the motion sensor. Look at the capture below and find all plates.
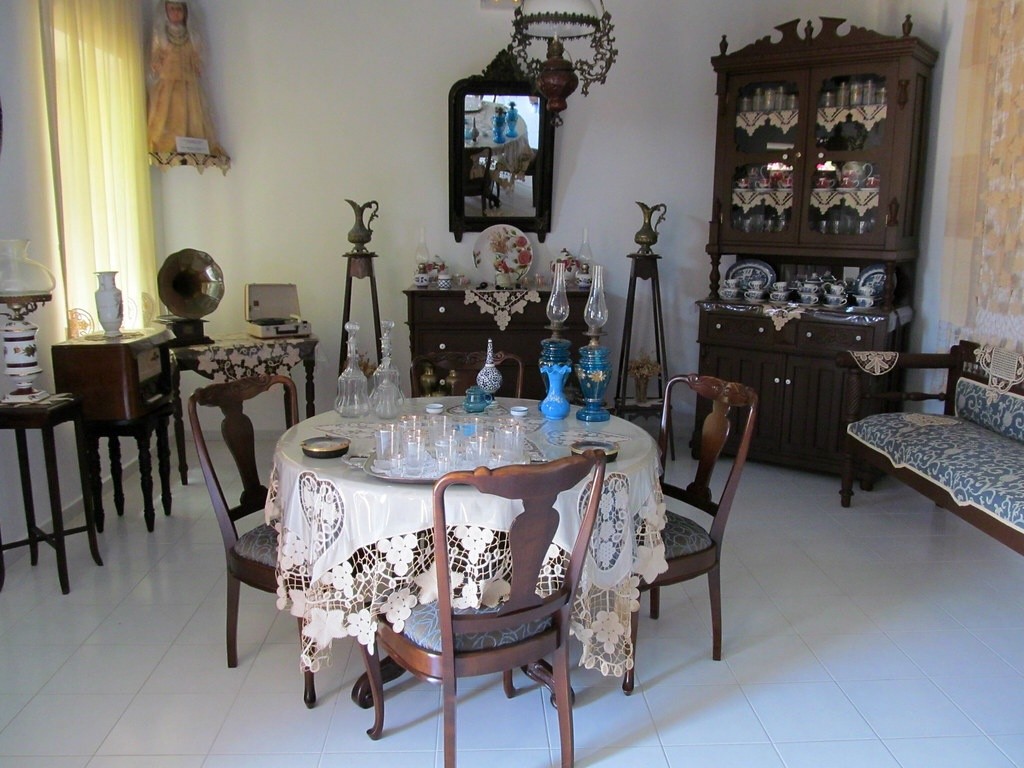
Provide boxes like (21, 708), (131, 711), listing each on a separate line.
(720, 260), (776, 299)
(719, 298), (876, 312)
(854, 262), (908, 303)
(446, 403), (510, 416)
(733, 188), (878, 191)
(474, 224), (533, 283)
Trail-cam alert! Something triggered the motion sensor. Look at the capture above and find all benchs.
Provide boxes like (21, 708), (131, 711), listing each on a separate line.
(835, 338), (1024, 555)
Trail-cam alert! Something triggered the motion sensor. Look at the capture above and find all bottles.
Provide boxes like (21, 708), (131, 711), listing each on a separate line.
(496, 254), (511, 290)
(335, 321), (405, 417)
(516, 273), (530, 289)
(577, 263), (592, 288)
(415, 263), (428, 285)
(477, 339), (502, 403)
(469, 117), (479, 142)
(741, 80), (887, 110)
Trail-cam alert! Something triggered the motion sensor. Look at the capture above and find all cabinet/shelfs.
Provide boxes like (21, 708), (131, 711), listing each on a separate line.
(689, 15), (939, 508)
(402, 280), (604, 406)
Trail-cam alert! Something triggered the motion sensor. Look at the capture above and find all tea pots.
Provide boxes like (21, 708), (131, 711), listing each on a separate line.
(760, 160), (874, 188)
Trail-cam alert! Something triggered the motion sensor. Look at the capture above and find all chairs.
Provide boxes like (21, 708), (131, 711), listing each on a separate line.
(623, 374), (759, 696)
(362, 450), (606, 768)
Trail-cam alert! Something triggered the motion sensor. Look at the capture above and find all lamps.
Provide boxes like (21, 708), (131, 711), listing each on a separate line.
(0, 238), (56, 404)
(505, 0), (618, 128)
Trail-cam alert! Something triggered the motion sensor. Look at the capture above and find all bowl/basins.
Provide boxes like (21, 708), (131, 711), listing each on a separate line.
(510, 406), (528, 416)
(425, 404), (444, 413)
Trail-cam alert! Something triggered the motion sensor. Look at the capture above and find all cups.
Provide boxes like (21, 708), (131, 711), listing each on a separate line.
(825, 283), (847, 305)
(852, 293), (874, 307)
(744, 281), (763, 299)
(797, 284), (819, 303)
(746, 167), (759, 179)
(374, 413), (525, 477)
(437, 274), (452, 290)
(858, 286), (875, 297)
(535, 274), (543, 285)
(770, 282), (792, 300)
(463, 385), (492, 412)
(738, 177), (880, 188)
(718, 277), (738, 297)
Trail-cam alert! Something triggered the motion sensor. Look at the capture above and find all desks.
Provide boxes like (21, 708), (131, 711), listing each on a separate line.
(263, 396), (667, 741)
(187, 375), (388, 709)
(0, 394), (103, 595)
(83, 402), (172, 533)
(169, 332), (319, 487)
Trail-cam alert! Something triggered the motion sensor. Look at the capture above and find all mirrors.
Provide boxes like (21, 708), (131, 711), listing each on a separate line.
(448, 49), (555, 243)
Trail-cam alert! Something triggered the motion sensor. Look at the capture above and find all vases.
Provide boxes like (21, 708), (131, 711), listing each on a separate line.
(95, 271), (124, 338)
